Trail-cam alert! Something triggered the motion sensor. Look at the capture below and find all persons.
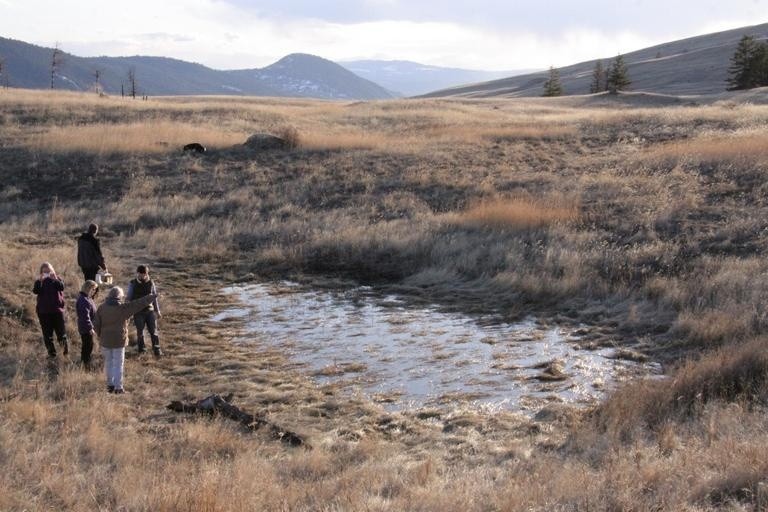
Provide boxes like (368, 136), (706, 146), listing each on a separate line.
(78, 222), (108, 291)
(92, 285), (164, 394)
(123, 264), (165, 358)
(75, 278), (98, 365)
(32, 262), (70, 361)
